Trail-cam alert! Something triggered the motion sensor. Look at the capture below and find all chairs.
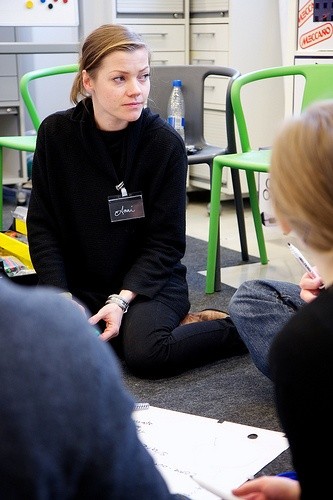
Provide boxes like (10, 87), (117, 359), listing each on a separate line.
(204, 63), (333, 293)
(0, 64), (82, 232)
(144, 65), (250, 292)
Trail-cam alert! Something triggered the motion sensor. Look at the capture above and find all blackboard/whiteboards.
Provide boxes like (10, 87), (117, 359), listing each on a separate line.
(258, 147), (276, 226)
(0, 1), (79, 27)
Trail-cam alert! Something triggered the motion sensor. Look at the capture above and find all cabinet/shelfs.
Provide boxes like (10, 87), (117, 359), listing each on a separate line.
(0, 0), (333, 217)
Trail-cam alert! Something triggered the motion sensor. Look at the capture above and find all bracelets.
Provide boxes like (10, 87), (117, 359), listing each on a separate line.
(106, 294), (129, 314)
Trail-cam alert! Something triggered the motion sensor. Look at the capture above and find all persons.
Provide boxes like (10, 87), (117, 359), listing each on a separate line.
(0, 99), (333, 500)
(0, 24), (250, 381)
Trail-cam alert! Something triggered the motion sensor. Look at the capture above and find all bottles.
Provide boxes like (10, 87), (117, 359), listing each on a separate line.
(168, 81), (185, 140)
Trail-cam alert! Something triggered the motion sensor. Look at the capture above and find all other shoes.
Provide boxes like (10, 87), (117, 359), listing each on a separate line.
(178, 308), (230, 326)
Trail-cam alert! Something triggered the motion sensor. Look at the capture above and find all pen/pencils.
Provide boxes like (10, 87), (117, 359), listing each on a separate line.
(287, 242), (325, 290)
(189, 475), (238, 500)
